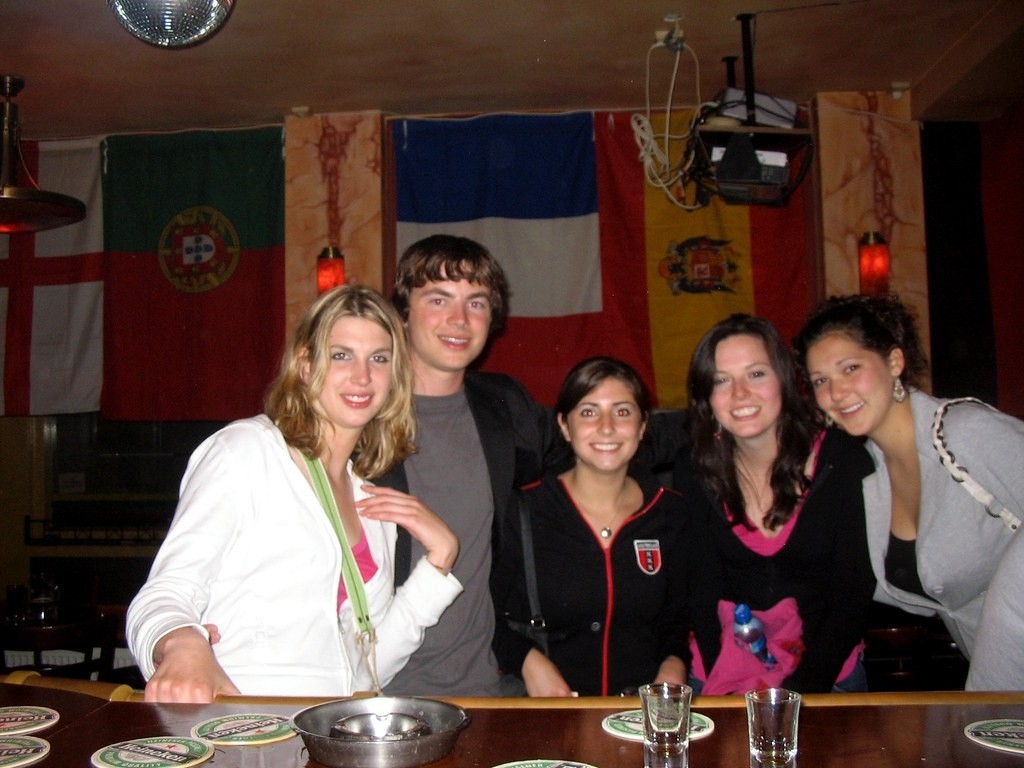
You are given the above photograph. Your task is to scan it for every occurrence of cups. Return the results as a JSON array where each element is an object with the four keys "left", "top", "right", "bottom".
[
  {"left": 745, "top": 687, "right": 801, "bottom": 768},
  {"left": 638, "top": 683, "right": 692, "bottom": 768}
]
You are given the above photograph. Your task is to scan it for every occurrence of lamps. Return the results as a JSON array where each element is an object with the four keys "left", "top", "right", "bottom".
[{"left": 0, "top": 75, "right": 86, "bottom": 235}]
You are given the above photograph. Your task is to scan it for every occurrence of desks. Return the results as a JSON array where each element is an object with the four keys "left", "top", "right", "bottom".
[{"left": 0, "top": 668, "right": 1024, "bottom": 768}]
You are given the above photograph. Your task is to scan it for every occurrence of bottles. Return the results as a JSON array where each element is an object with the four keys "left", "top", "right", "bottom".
[{"left": 732, "top": 604, "right": 769, "bottom": 662}]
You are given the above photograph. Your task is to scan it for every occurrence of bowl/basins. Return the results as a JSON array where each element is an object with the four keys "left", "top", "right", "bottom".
[{"left": 289, "top": 695, "right": 471, "bottom": 768}]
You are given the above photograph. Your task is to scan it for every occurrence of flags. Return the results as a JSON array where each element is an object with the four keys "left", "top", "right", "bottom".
[
  {"left": 0, "top": 120, "right": 286, "bottom": 422},
  {"left": 383, "top": 105, "right": 825, "bottom": 413}
]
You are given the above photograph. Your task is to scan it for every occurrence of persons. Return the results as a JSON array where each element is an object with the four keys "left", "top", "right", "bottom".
[
  {"left": 354, "top": 233, "right": 555, "bottom": 696},
  {"left": 487, "top": 356, "right": 711, "bottom": 698},
  {"left": 793, "top": 292, "right": 1024, "bottom": 694},
  {"left": 673, "top": 313, "right": 869, "bottom": 699},
  {"left": 123, "top": 283, "right": 467, "bottom": 705}
]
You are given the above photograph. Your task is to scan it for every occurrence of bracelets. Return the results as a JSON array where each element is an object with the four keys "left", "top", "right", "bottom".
[{"left": 429, "top": 561, "right": 455, "bottom": 573}]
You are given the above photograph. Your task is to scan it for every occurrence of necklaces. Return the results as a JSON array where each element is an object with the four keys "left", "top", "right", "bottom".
[
  {"left": 571, "top": 472, "right": 633, "bottom": 540},
  {"left": 732, "top": 443, "right": 775, "bottom": 516}
]
